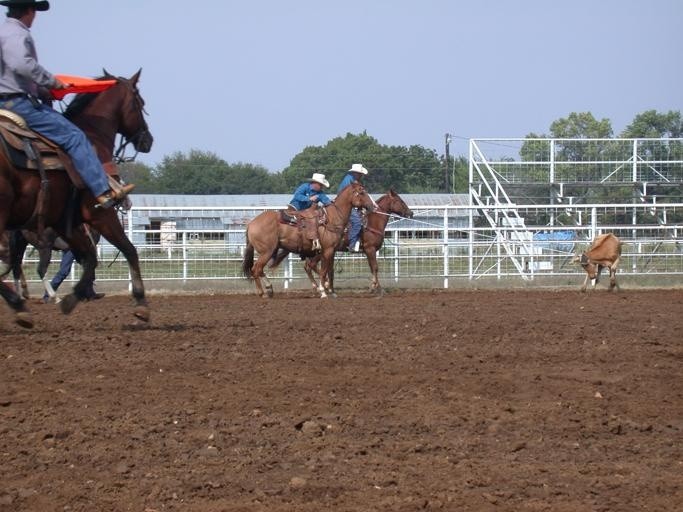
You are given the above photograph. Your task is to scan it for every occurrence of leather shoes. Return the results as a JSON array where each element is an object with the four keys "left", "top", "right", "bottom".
[
  {"left": 98, "top": 184, "right": 135, "bottom": 208},
  {"left": 90, "top": 293, "right": 104, "bottom": 300}
]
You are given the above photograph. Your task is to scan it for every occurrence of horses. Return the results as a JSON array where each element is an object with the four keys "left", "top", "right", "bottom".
[
  {"left": 299, "top": 187, "right": 416, "bottom": 297},
  {"left": 239, "top": 180, "right": 379, "bottom": 300},
  {"left": 0, "top": 67, "right": 154, "bottom": 326},
  {"left": 569, "top": 231, "right": 628, "bottom": 292}
]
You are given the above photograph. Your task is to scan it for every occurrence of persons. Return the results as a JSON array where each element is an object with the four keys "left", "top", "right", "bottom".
[
  {"left": 335, "top": 163, "right": 369, "bottom": 252},
  {"left": 287, "top": 173, "right": 333, "bottom": 210},
  {"left": 0, "top": 0, "right": 136, "bottom": 209},
  {"left": 39, "top": 239, "right": 108, "bottom": 304}
]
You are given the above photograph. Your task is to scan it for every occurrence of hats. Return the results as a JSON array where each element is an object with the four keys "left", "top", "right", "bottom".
[
  {"left": 1, "top": 0, "right": 50, "bottom": 12},
  {"left": 305, "top": 174, "right": 330, "bottom": 188},
  {"left": 348, "top": 164, "right": 368, "bottom": 174}
]
